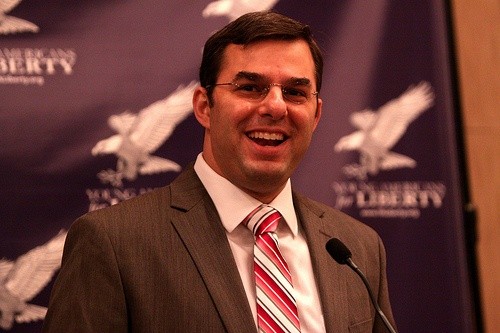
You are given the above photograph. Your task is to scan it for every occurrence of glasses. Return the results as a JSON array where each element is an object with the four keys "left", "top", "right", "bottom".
[{"left": 205, "top": 76, "right": 320, "bottom": 105}]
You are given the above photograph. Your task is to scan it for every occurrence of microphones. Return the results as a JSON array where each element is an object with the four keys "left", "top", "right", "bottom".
[{"left": 325, "top": 238, "right": 397, "bottom": 333}]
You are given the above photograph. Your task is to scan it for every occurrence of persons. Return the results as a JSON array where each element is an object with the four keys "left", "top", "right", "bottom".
[{"left": 43, "top": 11, "right": 397, "bottom": 333}]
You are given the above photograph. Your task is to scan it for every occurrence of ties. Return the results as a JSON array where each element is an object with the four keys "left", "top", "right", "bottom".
[{"left": 242, "top": 204, "right": 301, "bottom": 333}]
